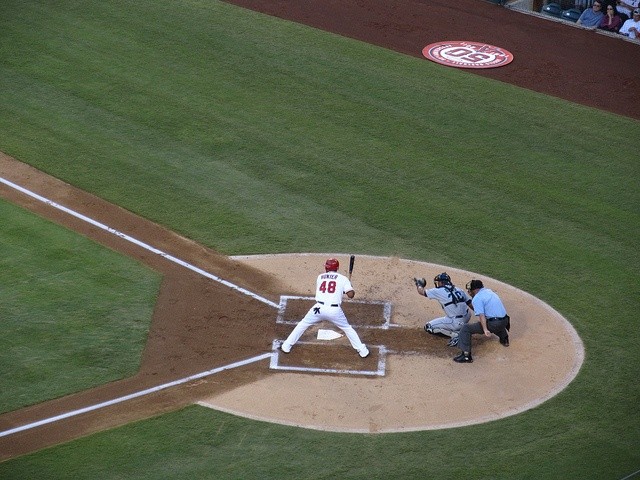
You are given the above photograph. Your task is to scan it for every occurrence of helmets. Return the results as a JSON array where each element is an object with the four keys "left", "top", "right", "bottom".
[
  {"left": 325, "top": 258, "right": 339, "bottom": 272},
  {"left": 434, "top": 272, "right": 451, "bottom": 288},
  {"left": 466, "top": 280, "right": 483, "bottom": 290}
]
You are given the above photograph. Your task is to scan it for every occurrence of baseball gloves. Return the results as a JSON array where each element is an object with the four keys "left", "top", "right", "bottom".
[{"left": 414, "top": 277, "right": 426, "bottom": 287}]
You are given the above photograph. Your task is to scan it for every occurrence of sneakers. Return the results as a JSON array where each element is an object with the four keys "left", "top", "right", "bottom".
[
  {"left": 447, "top": 337, "right": 459, "bottom": 347},
  {"left": 454, "top": 353, "right": 473, "bottom": 363},
  {"left": 275, "top": 340, "right": 290, "bottom": 353},
  {"left": 500, "top": 338, "right": 509, "bottom": 347},
  {"left": 360, "top": 344, "right": 369, "bottom": 356}
]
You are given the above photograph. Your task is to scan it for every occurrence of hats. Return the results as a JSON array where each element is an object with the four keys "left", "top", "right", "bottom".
[{"left": 633, "top": 8, "right": 640, "bottom": 15}]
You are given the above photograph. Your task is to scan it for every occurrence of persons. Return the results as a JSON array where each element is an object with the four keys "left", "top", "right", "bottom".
[
  {"left": 414, "top": 272, "right": 474, "bottom": 347},
  {"left": 618, "top": 8, "right": 640, "bottom": 40},
  {"left": 454, "top": 280, "right": 510, "bottom": 363},
  {"left": 576, "top": 0, "right": 604, "bottom": 29},
  {"left": 281, "top": 258, "right": 370, "bottom": 358},
  {"left": 600, "top": 2, "right": 622, "bottom": 33},
  {"left": 614, "top": 0, "right": 640, "bottom": 22}
]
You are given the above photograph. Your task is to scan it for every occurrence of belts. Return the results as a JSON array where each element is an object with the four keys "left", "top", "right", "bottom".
[
  {"left": 456, "top": 309, "right": 468, "bottom": 318},
  {"left": 486, "top": 314, "right": 508, "bottom": 321},
  {"left": 318, "top": 301, "right": 338, "bottom": 306}
]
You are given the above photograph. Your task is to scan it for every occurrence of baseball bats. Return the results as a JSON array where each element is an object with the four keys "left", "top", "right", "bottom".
[{"left": 348, "top": 255, "right": 355, "bottom": 282}]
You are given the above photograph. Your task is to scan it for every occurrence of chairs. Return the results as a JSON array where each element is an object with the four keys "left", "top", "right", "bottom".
[
  {"left": 541, "top": 3, "right": 562, "bottom": 18},
  {"left": 562, "top": 8, "right": 581, "bottom": 22}
]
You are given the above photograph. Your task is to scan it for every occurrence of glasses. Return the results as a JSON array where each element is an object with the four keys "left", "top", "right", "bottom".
[
  {"left": 593, "top": 5, "right": 601, "bottom": 7},
  {"left": 607, "top": 8, "right": 613, "bottom": 10}
]
